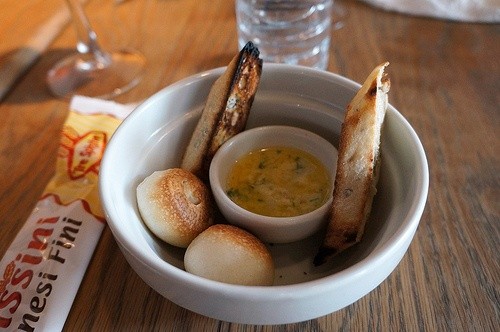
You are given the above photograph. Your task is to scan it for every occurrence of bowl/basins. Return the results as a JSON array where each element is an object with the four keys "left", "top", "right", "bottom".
[
  {"left": 210, "top": 124, "right": 341, "bottom": 246},
  {"left": 96, "top": 62, "right": 430, "bottom": 325}
]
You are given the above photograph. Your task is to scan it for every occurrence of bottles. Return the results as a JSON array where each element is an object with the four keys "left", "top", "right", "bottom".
[{"left": 236, "top": 0, "right": 333, "bottom": 71}]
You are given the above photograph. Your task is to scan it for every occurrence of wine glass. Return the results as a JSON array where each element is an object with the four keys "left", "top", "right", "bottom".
[{"left": 45, "top": 0, "right": 150, "bottom": 102}]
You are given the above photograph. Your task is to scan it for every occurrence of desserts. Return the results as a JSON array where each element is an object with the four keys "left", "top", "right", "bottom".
[
  {"left": 136, "top": 168, "right": 217, "bottom": 247},
  {"left": 184, "top": 224, "right": 274, "bottom": 287}
]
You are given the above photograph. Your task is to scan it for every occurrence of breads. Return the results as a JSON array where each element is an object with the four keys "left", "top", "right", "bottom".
[
  {"left": 313, "top": 61, "right": 390, "bottom": 266},
  {"left": 180, "top": 41, "right": 263, "bottom": 182}
]
